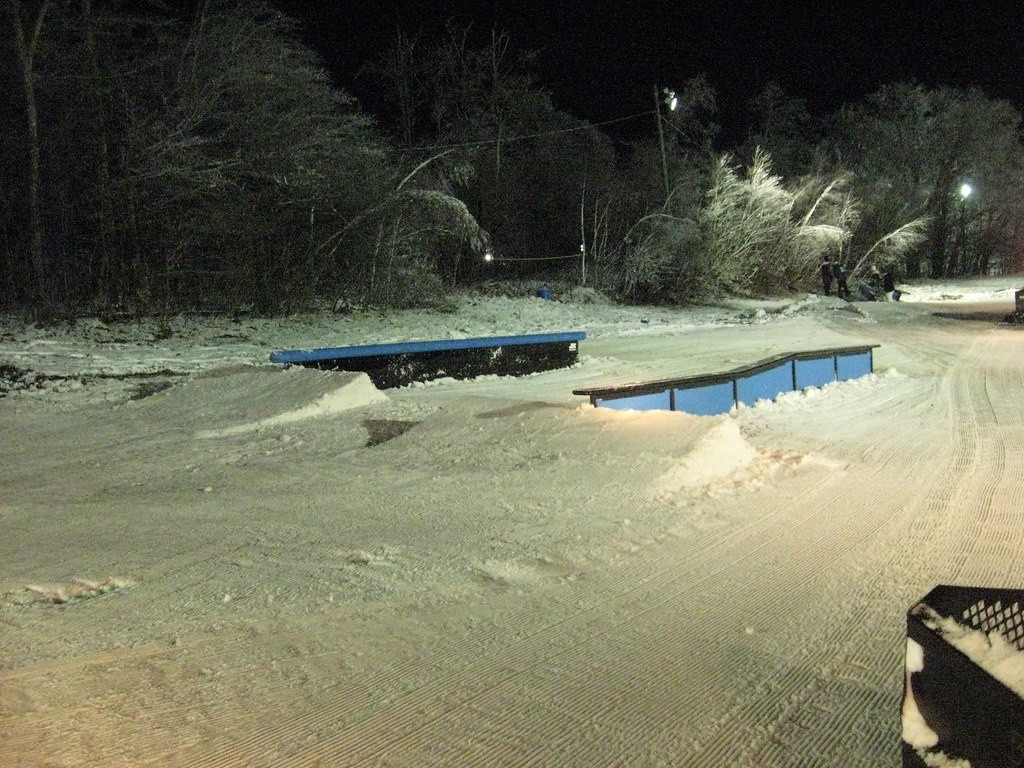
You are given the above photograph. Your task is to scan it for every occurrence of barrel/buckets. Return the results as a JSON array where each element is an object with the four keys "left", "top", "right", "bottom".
[
  {"left": 892, "top": 290, "right": 902, "bottom": 301},
  {"left": 538, "top": 288, "right": 553, "bottom": 300}
]
